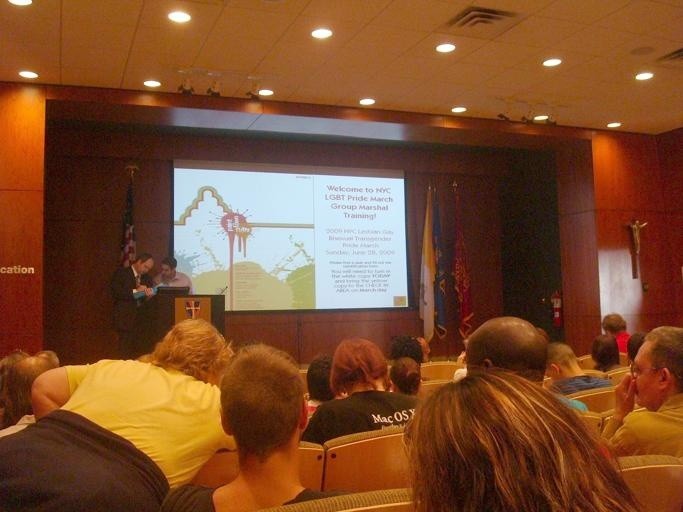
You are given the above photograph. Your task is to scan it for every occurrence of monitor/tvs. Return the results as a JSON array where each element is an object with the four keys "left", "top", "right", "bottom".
[{"left": 157, "top": 286, "right": 190, "bottom": 294}]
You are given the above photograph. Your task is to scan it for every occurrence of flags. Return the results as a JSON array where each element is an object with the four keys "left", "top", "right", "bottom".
[
  {"left": 121, "top": 168, "right": 140, "bottom": 272},
  {"left": 454, "top": 182, "right": 474, "bottom": 342},
  {"left": 420, "top": 186, "right": 436, "bottom": 350},
  {"left": 436, "top": 184, "right": 447, "bottom": 344}
]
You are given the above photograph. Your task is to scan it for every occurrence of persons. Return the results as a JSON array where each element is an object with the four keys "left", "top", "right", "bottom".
[
  {"left": 399, "top": 368, "right": 654, "bottom": 512},
  {"left": 601, "top": 327, "right": 683, "bottom": 457},
  {"left": 1, "top": 316, "right": 237, "bottom": 510},
  {"left": 113, "top": 253, "right": 190, "bottom": 360},
  {"left": 1, "top": 315, "right": 646, "bottom": 448},
  {"left": 160, "top": 341, "right": 351, "bottom": 512}
]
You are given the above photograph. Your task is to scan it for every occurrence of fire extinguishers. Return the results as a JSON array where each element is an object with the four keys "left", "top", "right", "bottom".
[{"left": 552, "top": 288, "right": 562, "bottom": 328}]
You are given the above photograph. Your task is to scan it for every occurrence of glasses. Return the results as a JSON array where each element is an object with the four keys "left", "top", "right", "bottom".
[{"left": 630, "top": 361, "right": 657, "bottom": 379}]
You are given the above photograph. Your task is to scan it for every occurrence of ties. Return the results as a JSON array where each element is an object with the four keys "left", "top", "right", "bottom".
[{"left": 136, "top": 275, "right": 139, "bottom": 287}]
